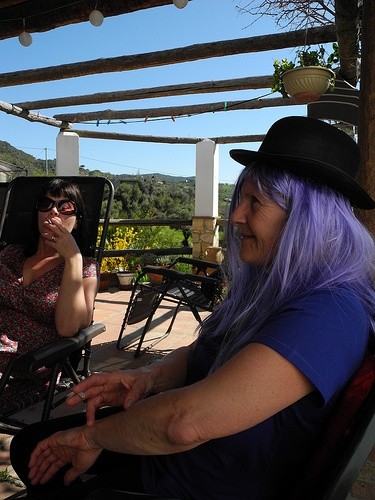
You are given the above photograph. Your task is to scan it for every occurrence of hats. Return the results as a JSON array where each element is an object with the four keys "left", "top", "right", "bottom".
[{"left": 229, "top": 116, "right": 375, "bottom": 210}]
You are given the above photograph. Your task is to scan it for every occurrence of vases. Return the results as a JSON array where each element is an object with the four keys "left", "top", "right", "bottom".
[
  {"left": 100, "top": 277, "right": 110, "bottom": 290},
  {"left": 116, "top": 273, "right": 134, "bottom": 285}
]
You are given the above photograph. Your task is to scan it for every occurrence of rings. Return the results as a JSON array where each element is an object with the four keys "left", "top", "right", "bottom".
[{"left": 51, "top": 235, "right": 55, "bottom": 241}]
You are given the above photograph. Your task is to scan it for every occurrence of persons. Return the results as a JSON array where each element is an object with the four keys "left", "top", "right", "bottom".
[
  {"left": 0, "top": 178, "right": 101, "bottom": 420},
  {"left": 65, "top": 116, "right": 375, "bottom": 500}
]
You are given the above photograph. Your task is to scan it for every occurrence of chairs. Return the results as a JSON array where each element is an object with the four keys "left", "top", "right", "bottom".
[{"left": 0, "top": 174, "right": 375, "bottom": 500}]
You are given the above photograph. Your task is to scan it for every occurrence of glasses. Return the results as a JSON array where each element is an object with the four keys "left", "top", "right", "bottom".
[{"left": 36, "top": 195, "right": 78, "bottom": 216}]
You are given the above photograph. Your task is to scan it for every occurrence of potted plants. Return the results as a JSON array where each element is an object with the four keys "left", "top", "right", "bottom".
[
  {"left": 139, "top": 250, "right": 172, "bottom": 284},
  {"left": 271, "top": 45, "right": 337, "bottom": 104}
]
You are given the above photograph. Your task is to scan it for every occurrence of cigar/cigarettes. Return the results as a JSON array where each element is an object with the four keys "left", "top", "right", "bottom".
[
  {"left": 44, "top": 217, "right": 53, "bottom": 224},
  {"left": 69, "top": 383, "right": 85, "bottom": 399}
]
